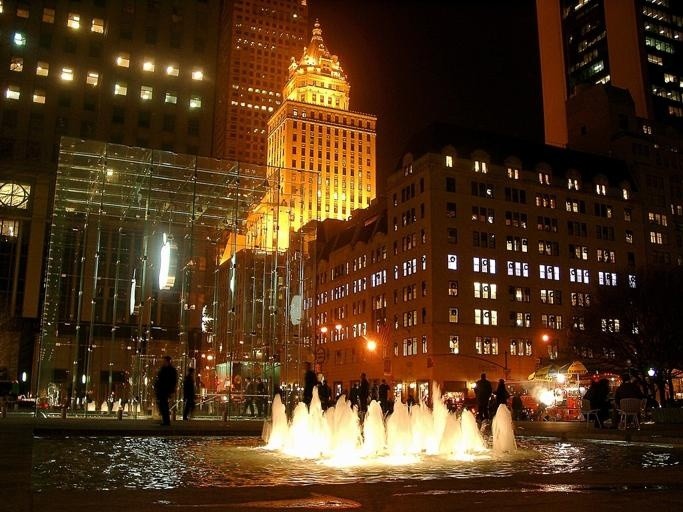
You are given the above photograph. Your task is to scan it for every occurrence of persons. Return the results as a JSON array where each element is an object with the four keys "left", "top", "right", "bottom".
[
  {"left": 614, "top": 372, "right": 641, "bottom": 400},
  {"left": 151, "top": 355, "right": 178, "bottom": 424},
  {"left": 473, "top": 372, "right": 489, "bottom": 419},
  {"left": 181, "top": 366, "right": 197, "bottom": 421},
  {"left": 583, "top": 377, "right": 615, "bottom": 431},
  {"left": 491, "top": 376, "right": 509, "bottom": 405},
  {"left": 241, "top": 361, "right": 416, "bottom": 421},
  {"left": 511, "top": 393, "right": 524, "bottom": 420}
]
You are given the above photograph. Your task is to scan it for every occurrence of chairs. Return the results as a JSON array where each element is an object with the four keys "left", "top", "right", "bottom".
[{"left": 577, "top": 397, "right": 646, "bottom": 433}]
